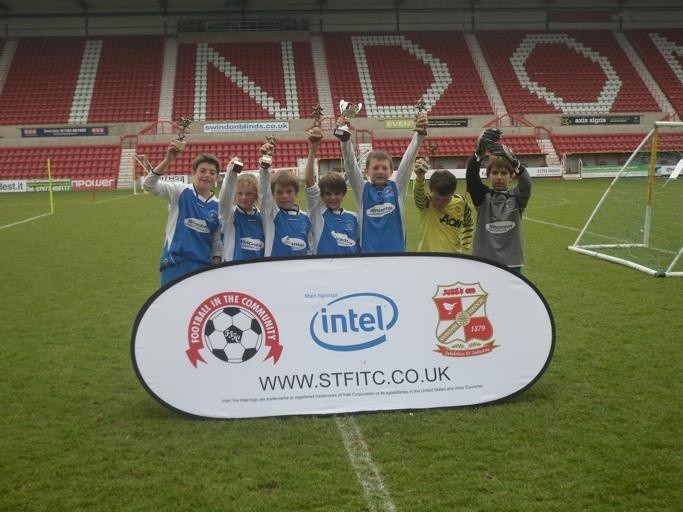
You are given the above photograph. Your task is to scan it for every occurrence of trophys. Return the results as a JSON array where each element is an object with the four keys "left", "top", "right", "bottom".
[
  {"left": 230, "top": 156, "right": 243, "bottom": 174},
  {"left": 310, "top": 104, "right": 324, "bottom": 141},
  {"left": 177, "top": 116, "right": 193, "bottom": 151},
  {"left": 333, "top": 99, "right": 362, "bottom": 142},
  {"left": 414, "top": 144, "right": 436, "bottom": 173},
  {"left": 414, "top": 97, "right": 427, "bottom": 136},
  {"left": 259, "top": 134, "right": 278, "bottom": 169},
  {"left": 489, "top": 154, "right": 502, "bottom": 162}
]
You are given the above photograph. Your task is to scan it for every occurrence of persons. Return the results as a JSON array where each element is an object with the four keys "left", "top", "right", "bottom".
[
  {"left": 305, "top": 128, "right": 359, "bottom": 255},
  {"left": 413, "top": 159, "right": 474, "bottom": 254},
  {"left": 258, "top": 143, "right": 312, "bottom": 257},
  {"left": 466, "top": 128, "right": 532, "bottom": 274},
  {"left": 336, "top": 113, "right": 429, "bottom": 255},
  {"left": 142, "top": 141, "right": 223, "bottom": 291},
  {"left": 218, "top": 156, "right": 265, "bottom": 262}
]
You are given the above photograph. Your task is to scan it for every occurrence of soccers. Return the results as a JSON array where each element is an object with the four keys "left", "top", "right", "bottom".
[{"left": 205, "top": 306, "right": 264, "bottom": 363}]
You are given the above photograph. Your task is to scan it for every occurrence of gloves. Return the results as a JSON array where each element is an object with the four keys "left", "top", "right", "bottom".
[
  {"left": 475, "top": 128, "right": 503, "bottom": 158},
  {"left": 488, "top": 143, "right": 520, "bottom": 170}
]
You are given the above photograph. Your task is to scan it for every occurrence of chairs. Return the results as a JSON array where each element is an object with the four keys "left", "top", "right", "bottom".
[
  {"left": 171, "top": 40, "right": 321, "bottom": 122},
  {"left": 0, "top": 144, "right": 121, "bottom": 179},
  {"left": 548, "top": 131, "right": 683, "bottom": 155},
  {"left": 321, "top": 29, "right": 495, "bottom": 119},
  {"left": 133, "top": 136, "right": 344, "bottom": 177},
  {"left": 0, "top": 33, "right": 166, "bottom": 124},
  {"left": 475, "top": 28, "right": 661, "bottom": 116},
  {"left": 626, "top": 27, "right": 683, "bottom": 121},
  {"left": 372, "top": 135, "right": 542, "bottom": 157}
]
